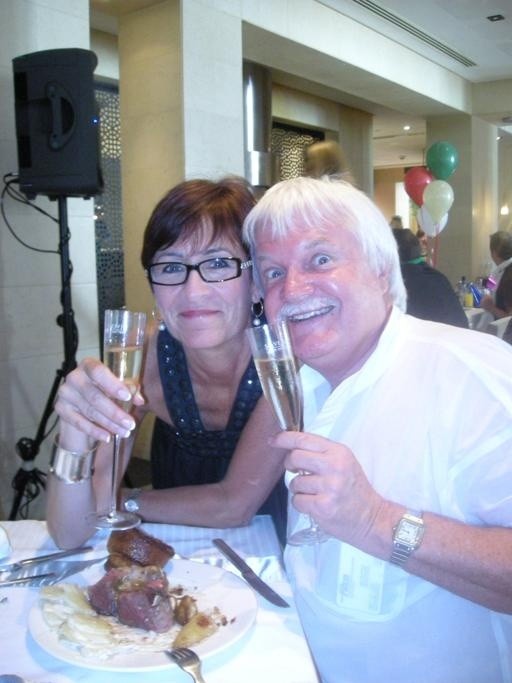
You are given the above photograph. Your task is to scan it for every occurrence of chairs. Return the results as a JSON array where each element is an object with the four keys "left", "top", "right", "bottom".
[{"left": 487, "top": 315, "right": 512, "bottom": 345}]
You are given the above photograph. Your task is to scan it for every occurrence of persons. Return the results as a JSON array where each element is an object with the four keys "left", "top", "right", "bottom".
[
  {"left": 43, "top": 174, "right": 296, "bottom": 552},
  {"left": 394, "top": 226, "right": 470, "bottom": 332},
  {"left": 476, "top": 231, "right": 511, "bottom": 346},
  {"left": 239, "top": 174, "right": 511, "bottom": 683}
]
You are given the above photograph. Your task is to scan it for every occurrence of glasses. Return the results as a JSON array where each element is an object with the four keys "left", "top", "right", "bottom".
[{"left": 145, "top": 256, "right": 256, "bottom": 286}]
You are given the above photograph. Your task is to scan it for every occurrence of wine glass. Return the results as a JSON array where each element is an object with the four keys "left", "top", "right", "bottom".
[
  {"left": 83, "top": 308, "right": 147, "bottom": 530},
  {"left": 245, "top": 318, "right": 331, "bottom": 547}
]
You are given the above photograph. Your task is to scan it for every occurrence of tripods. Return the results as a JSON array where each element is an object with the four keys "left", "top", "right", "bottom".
[{"left": 9, "top": 202, "right": 80, "bottom": 519}]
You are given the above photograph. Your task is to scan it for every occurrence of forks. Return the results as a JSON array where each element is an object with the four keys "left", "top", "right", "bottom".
[{"left": 164, "top": 646, "right": 206, "bottom": 683}]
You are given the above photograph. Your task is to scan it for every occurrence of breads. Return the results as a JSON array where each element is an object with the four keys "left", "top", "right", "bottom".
[{"left": 39, "top": 581, "right": 120, "bottom": 660}]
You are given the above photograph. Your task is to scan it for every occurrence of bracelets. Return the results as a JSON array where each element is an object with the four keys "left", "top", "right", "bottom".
[{"left": 47, "top": 431, "right": 98, "bottom": 482}]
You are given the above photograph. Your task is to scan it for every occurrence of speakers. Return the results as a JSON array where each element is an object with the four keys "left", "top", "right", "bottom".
[{"left": 12, "top": 48, "right": 107, "bottom": 200}]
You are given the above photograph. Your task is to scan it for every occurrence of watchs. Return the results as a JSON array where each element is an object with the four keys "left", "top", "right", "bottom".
[
  {"left": 391, "top": 508, "right": 425, "bottom": 572},
  {"left": 124, "top": 485, "right": 142, "bottom": 513}
]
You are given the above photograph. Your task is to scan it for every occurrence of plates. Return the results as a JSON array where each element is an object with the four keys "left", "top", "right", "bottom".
[{"left": 27, "top": 555, "right": 258, "bottom": 672}]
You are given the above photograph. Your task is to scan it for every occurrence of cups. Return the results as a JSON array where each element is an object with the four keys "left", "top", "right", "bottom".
[{"left": 464, "top": 287, "right": 473, "bottom": 308}]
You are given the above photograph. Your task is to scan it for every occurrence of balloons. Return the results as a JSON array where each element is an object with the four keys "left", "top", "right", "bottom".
[
  {"left": 421, "top": 178, "right": 455, "bottom": 225},
  {"left": 414, "top": 205, "right": 449, "bottom": 236},
  {"left": 405, "top": 166, "right": 434, "bottom": 208},
  {"left": 425, "top": 139, "right": 459, "bottom": 181}
]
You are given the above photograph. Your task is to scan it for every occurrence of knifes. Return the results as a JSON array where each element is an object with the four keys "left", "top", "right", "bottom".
[
  {"left": 0, "top": 546, "right": 93, "bottom": 575},
  {"left": 212, "top": 538, "right": 290, "bottom": 608}
]
